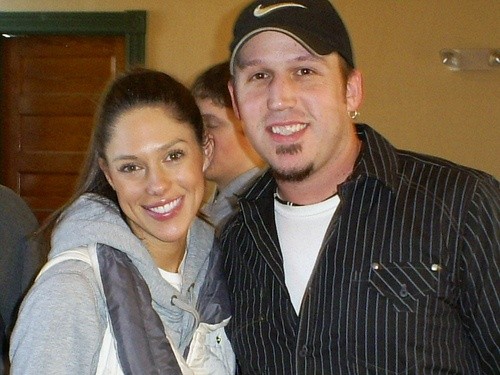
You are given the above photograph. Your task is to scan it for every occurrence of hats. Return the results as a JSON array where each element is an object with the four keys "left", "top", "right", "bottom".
[{"left": 229, "top": 0, "right": 354, "bottom": 76}]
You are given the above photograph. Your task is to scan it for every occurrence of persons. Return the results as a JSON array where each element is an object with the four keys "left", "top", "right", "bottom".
[
  {"left": 190, "top": 62, "right": 269, "bottom": 226},
  {"left": 217, "top": 0, "right": 500, "bottom": 375},
  {"left": 0, "top": 184, "right": 47, "bottom": 375},
  {"left": 9, "top": 70, "right": 216, "bottom": 374}
]
